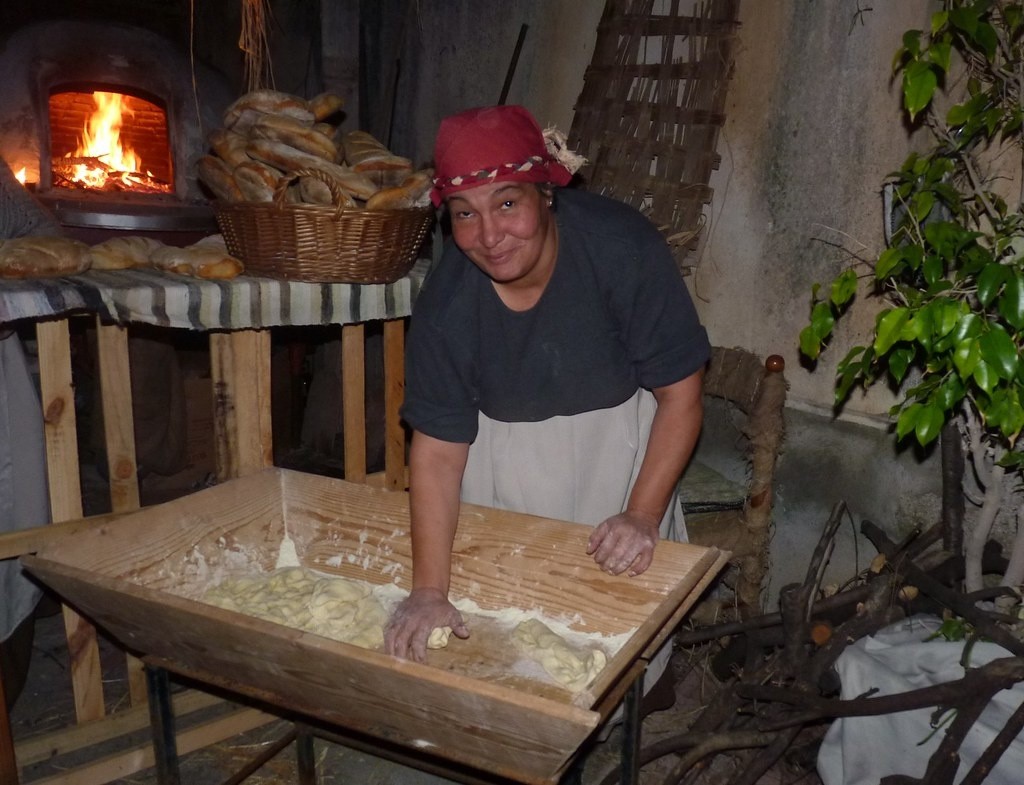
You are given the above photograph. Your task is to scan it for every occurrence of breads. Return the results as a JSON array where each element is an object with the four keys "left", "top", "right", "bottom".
[{"left": 196, "top": 90, "right": 432, "bottom": 213}]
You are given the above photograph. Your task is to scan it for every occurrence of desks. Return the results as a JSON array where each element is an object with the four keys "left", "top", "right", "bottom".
[{"left": 141, "top": 660, "right": 644, "bottom": 785}]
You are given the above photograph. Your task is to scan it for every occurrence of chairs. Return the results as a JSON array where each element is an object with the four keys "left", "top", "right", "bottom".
[{"left": 676, "top": 348, "right": 782, "bottom": 612}]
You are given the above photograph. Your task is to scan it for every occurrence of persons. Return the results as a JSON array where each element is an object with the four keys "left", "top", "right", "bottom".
[
  {"left": 382, "top": 106, "right": 711, "bottom": 724},
  {"left": 1, "top": 151, "right": 72, "bottom": 714}
]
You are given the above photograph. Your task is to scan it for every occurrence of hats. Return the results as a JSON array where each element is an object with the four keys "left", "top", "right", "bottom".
[{"left": 424, "top": 106, "right": 573, "bottom": 206}]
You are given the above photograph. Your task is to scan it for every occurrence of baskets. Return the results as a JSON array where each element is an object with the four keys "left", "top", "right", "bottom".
[{"left": 208, "top": 168, "right": 438, "bottom": 284}]
